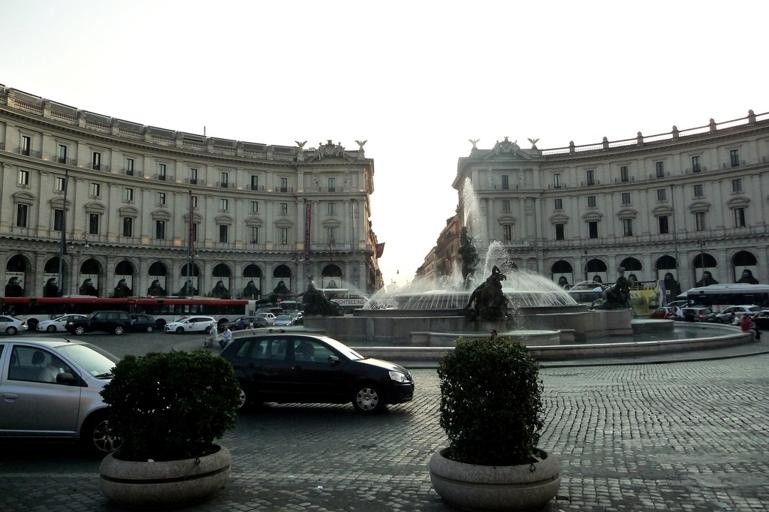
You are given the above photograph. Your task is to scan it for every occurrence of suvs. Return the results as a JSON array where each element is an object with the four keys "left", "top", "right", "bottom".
[{"left": 65, "top": 310, "right": 134, "bottom": 335}]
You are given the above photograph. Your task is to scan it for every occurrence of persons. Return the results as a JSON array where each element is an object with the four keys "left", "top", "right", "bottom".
[
  {"left": 730, "top": 312, "right": 763, "bottom": 342},
  {"left": 204, "top": 322, "right": 217, "bottom": 347},
  {"left": 218, "top": 326, "right": 233, "bottom": 348}
]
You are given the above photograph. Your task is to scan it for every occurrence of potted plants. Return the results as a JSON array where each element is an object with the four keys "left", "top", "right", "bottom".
[
  {"left": 96, "top": 347, "right": 245, "bottom": 508},
  {"left": 429, "top": 327, "right": 563, "bottom": 509}
]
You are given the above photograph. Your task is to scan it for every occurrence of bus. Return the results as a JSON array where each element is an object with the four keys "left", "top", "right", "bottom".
[
  {"left": 1, "top": 296, "right": 249, "bottom": 331},
  {"left": 687, "top": 284, "right": 769, "bottom": 308}
]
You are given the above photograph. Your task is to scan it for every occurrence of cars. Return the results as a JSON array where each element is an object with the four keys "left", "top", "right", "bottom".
[
  {"left": 0, "top": 338, "right": 124, "bottom": 455},
  {"left": 0, "top": 314, "right": 28, "bottom": 335},
  {"left": 655, "top": 300, "right": 769, "bottom": 328},
  {"left": 165, "top": 316, "right": 217, "bottom": 334},
  {"left": 37, "top": 314, "right": 86, "bottom": 333},
  {"left": 129, "top": 314, "right": 156, "bottom": 333},
  {"left": 221, "top": 335, "right": 414, "bottom": 414},
  {"left": 220, "top": 301, "right": 304, "bottom": 330}
]
